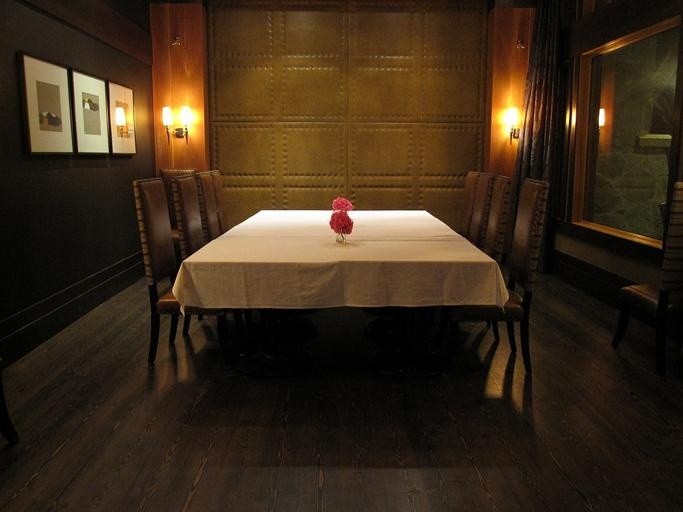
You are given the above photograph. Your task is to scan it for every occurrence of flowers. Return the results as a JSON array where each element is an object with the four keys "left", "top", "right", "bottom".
[{"left": 326, "top": 197, "right": 355, "bottom": 248}]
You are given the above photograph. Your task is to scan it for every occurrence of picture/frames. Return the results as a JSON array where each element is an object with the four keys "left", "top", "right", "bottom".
[
  {"left": 14, "top": 48, "right": 75, "bottom": 162},
  {"left": 106, "top": 78, "right": 136, "bottom": 161},
  {"left": 65, "top": 65, "right": 111, "bottom": 159}
]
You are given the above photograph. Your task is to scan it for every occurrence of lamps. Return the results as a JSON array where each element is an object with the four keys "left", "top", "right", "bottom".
[
  {"left": 499, "top": 104, "right": 523, "bottom": 146},
  {"left": 159, "top": 105, "right": 197, "bottom": 147}
]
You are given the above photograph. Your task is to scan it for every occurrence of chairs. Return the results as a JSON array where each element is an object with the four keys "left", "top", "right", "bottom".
[
  {"left": 610, "top": 180, "right": 683, "bottom": 376},
  {"left": 125, "top": 165, "right": 253, "bottom": 372},
  {"left": 413, "top": 168, "right": 550, "bottom": 377}
]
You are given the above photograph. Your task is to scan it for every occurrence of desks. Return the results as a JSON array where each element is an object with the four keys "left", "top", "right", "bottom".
[{"left": 160, "top": 207, "right": 510, "bottom": 389}]
